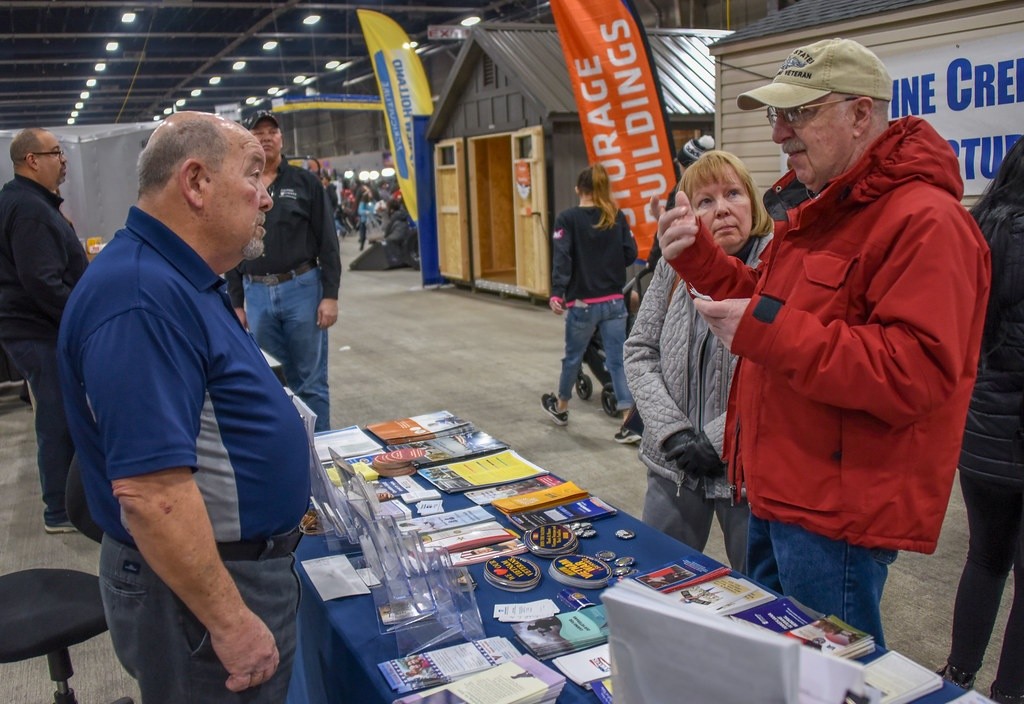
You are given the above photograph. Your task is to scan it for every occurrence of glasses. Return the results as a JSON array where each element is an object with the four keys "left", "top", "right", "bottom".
[
  {"left": 765, "top": 95, "right": 858, "bottom": 129},
  {"left": 24, "top": 150, "right": 63, "bottom": 161}
]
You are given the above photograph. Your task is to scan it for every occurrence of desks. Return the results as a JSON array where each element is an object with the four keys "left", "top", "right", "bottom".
[{"left": 286, "top": 410, "right": 1011, "bottom": 704}]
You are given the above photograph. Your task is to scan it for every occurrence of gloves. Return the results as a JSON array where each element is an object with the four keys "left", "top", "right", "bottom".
[{"left": 662, "top": 429, "right": 725, "bottom": 481}]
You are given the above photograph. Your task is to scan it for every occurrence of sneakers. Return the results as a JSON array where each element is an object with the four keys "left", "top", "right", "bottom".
[
  {"left": 541, "top": 393, "right": 568, "bottom": 426},
  {"left": 614, "top": 426, "right": 642, "bottom": 444},
  {"left": 44, "top": 521, "right": 78, "bottom": 533}
]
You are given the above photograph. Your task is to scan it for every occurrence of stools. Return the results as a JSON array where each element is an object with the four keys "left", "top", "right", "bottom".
[{"left": 0, "top": 567, "right": 133, "bottom": 704}]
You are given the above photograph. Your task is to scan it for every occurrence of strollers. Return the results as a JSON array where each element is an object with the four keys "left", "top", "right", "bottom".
[{"left": 561, "top": 268, "right": 657, "bottom": 416}]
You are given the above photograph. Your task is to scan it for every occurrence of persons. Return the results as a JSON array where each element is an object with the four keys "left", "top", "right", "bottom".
[
  {"left": 225, "top": 109, "right": 343, "bottom": 434},
  {"left": 623, "top": 148, "right": 775, "bottom": 576},
  {"left": 55, "top": 110, "right": 312, "bottom": 704},
  {"left": 647, "top": 135, "right": 715, "bottom": 271},
  {"left": 657, "top": 35, "right": 993, "bottom": 653},
  {"left": 324, "top": 176, "right": 346, "bottom": 239},
  {"left": 0, "top": 126, "right": 92, "bottom": 535},
  {"left": 339, "top": 181, "right": 402, "bottom": 231},
  {"left": 934, "top": 133, "right": 1024, "bottom": 704},
  {"left": 541, "top": 163, "right": 641, "bottom": 444},
  {"left": 629, "top": 266, "right": 655, "bottom": 316}
]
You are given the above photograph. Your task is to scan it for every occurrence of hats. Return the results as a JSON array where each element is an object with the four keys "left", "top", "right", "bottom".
[
  {"left": 678, "top": 134, "right": 714, "bottom": 168},
  {"left": 736, "top": 38, "right": 893, "bottom": 110},
  {"left": 241, "top": 103, "right": 279, "bottom": 131}
]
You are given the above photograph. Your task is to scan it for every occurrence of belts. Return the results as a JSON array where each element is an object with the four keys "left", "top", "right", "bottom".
[
  {"left": 214, "top": 530, "right": 304, "bottom": 561},
  {"left": 244, "top": 260, "right": 317, "bottom": 286}
]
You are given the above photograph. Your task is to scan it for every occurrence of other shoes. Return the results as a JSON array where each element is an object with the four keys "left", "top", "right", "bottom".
[
  {"left": 989, "top": 679, "right": 1024, "bottom": 704},
  {"left": 935, "top": 664, "right": 977, "bottom": 691}
]
